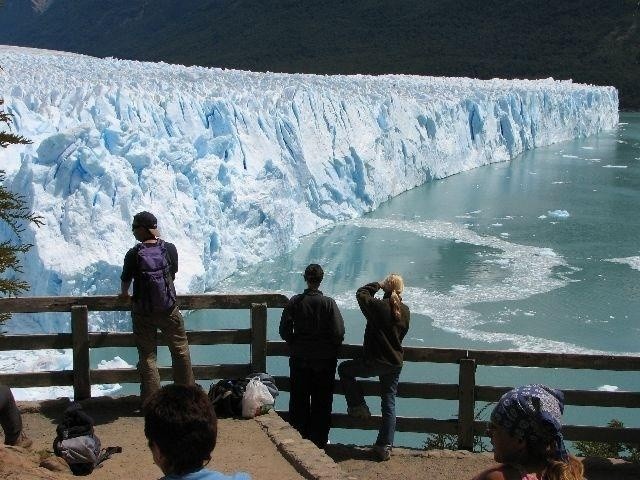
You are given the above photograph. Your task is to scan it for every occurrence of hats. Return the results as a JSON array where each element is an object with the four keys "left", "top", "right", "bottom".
[{"left": 134, "top": 212, "right": 162, "bottom": 238}]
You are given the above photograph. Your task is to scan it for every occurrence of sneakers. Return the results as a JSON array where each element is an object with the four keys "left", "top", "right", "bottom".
[
  {"left": 347, "top": 407, "right": 371, "bottom": 420},
  {"left": 371, "top": 444, "right": 393, "bottom": 461},
  {"left": 6, "top": 436, "right": 30, "bottom": 448}
]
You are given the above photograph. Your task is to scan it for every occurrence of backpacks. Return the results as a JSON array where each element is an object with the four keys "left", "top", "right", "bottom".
[{"left": 133, "top": 240, "right": 176, "bottom": 315}]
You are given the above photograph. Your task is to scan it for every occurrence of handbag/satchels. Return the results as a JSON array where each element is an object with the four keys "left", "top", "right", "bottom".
[
  {"left": 208, "top": 372, "right": 279, "bottom": 418},
  {"left": 54, "top": 398, "right": 100, "bottom": 476}
]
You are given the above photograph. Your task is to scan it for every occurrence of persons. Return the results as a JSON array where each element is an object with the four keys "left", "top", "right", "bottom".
[
  {"left": 119, "top": 211, "right": 202, "bottom": 401},
  {"left": 0, "top": 383, "right": 33, "bottom": 448},
  {"left": 472, "top": 384, "right": 587, "bottom": 480},
  {"left": 279, "top": 264, "right": 346, "bottom": 450},
  {"left": 338, "top": 274, "right": 411, "bottom": 459},
  {"left": 143, "top": 382, "right": 253, "bottom": 480}
]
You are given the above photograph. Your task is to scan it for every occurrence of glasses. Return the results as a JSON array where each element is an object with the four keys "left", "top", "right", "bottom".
[
  {"left": 132, "top": 224, "right": 139, "bottom": 228},
  {"left": 485, "top": 421, "right": 505, "bottom": 438}
]
